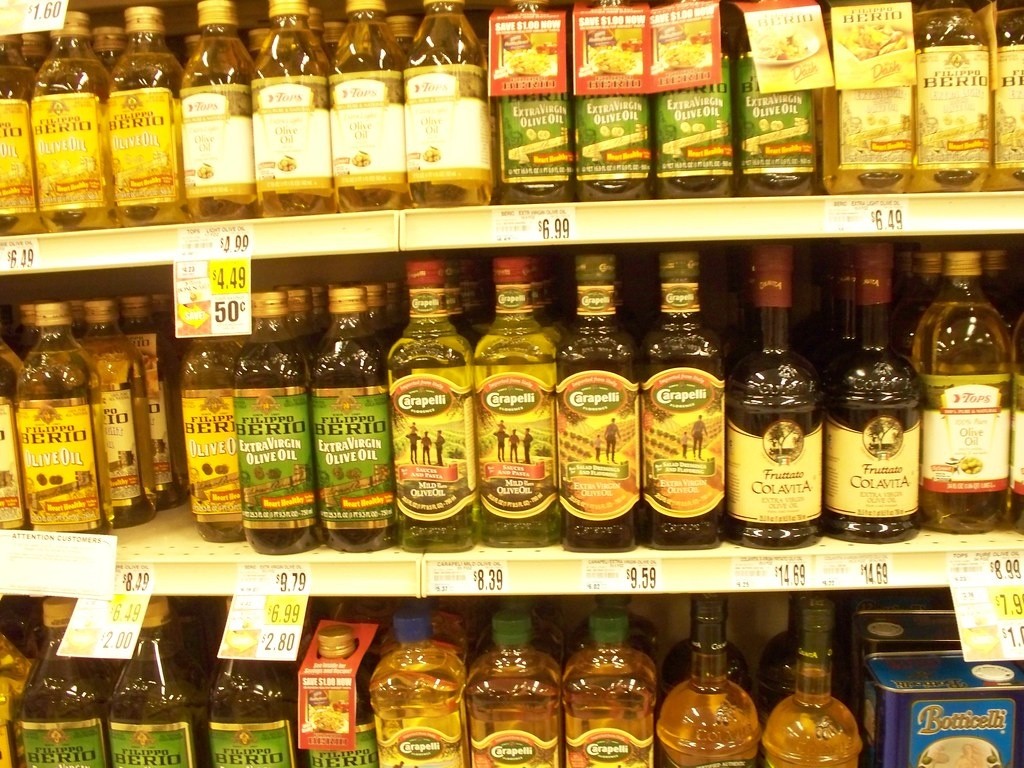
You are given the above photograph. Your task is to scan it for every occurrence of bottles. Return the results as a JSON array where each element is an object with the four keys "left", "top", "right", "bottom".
[{"left": 0, "top": 0, "right": 1024, "bottom": 768}]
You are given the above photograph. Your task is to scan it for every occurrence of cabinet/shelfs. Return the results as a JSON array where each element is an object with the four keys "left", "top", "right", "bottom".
[{"left": 0, "top": 0, "right": 1024, "bottom": 768}]
